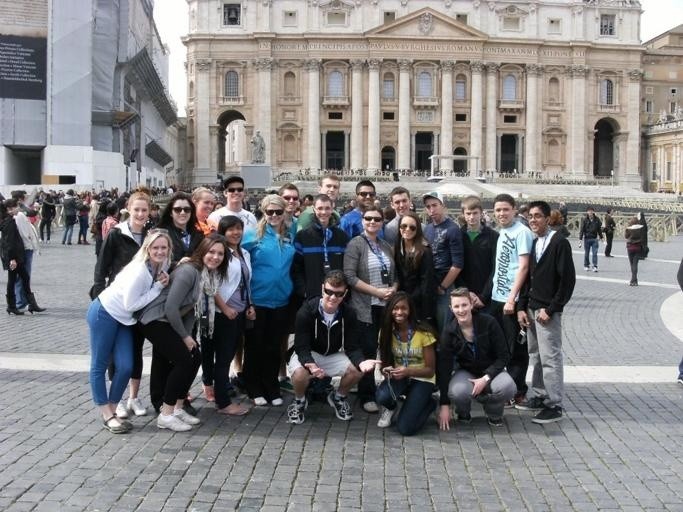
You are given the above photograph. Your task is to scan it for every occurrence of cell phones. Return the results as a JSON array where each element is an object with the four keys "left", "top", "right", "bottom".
[{"left": 385, "top": 369, "right": 393, "bottom": 373}]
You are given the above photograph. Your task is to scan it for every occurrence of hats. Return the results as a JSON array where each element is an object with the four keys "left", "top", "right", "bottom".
[
  {"left": 223, "top": 175, "right": 244, "bottom": 188},
  {"left": 421, "top": 191, "right": 443, "bottom": 203}
]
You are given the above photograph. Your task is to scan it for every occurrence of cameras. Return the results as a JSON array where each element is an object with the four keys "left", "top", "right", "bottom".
[{"left": 517, "top": 330, "right": 527, "bottom": 345}]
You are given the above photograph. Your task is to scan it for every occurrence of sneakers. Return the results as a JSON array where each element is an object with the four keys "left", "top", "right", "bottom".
[
  {"left": 101, "top": 373, "right": 354, "bottom": 435},
  {"left": 39, "top": 238, "right": 91, "bottom": 245},
  {"left": 349, "top": 382, "right": 562, "bottom": 427}
]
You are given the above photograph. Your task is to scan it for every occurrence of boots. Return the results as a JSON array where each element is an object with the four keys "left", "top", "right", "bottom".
[
  {"left": 25, "top": 292, "right": 47, "bottom": 314},
  {"left": 6, "top": 294, "right": 24, "bottom": 315}
]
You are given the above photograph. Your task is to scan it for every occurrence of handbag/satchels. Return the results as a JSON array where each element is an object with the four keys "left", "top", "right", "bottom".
[{"left": 642, "top": 245, "right": 649, "bottom": 258}]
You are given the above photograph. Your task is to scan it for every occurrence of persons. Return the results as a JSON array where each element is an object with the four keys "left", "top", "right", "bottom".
[
  {"left": 637, "top": 212, "right": 649, "bottom": 260},
  {"left": 1, "top": 172, "right": 575, "bottom": 437},
  {"left": 579, "top": 206, "right": 604, "bottom": 272},
  {"left": 676, "top": 259, "right": 683, "bottom": 386},
  {"left": 624, "top": 216, "right": 644, "bottom": 285},
  {"left": 604, "top": 208, "right": 616, "bottom": 257},
  {"left": 250, "top": 129, "right": 268, "bottom": 166}
]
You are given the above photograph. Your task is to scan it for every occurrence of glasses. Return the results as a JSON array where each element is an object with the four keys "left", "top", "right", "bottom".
[
  {"left": 527, "top": 213, "right": 546, "bottom": 220},
  {"left": 323, "top": 285, "right": 346, "bottom": 297},
  {"left": 148, "top": 188, "right": 417, "bottom": 233}
]
